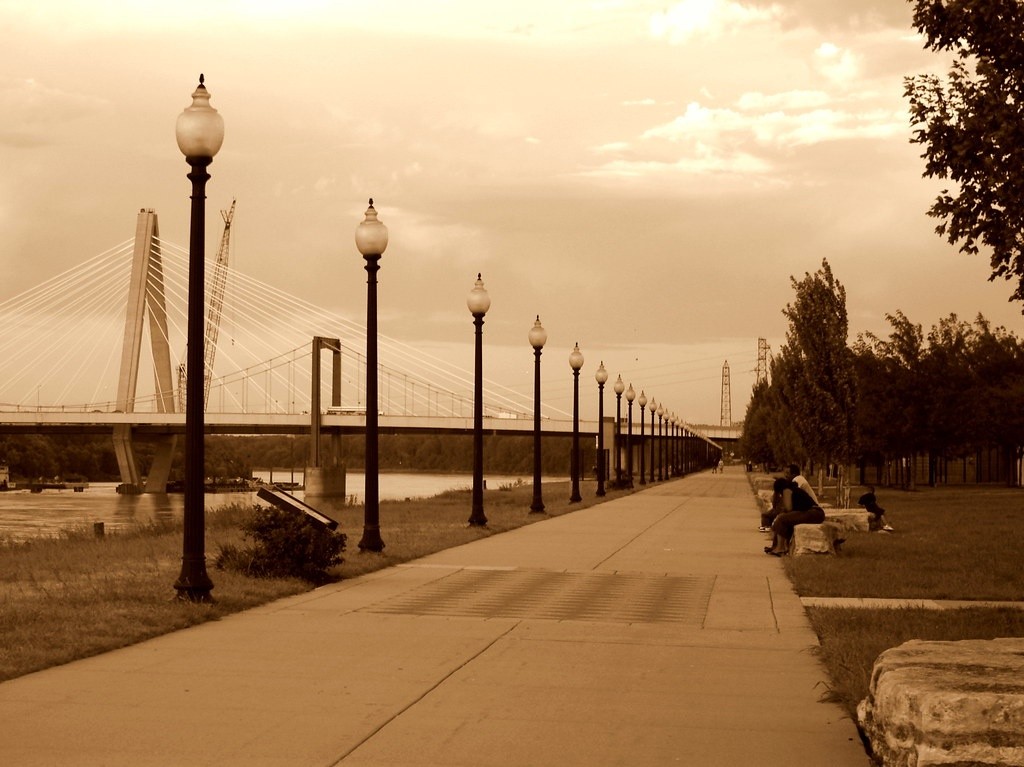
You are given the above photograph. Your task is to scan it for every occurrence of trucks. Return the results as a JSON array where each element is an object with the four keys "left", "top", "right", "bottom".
[
  {"left": 327, "top": 407, "right": 384, "bottom": 416},
  {"left": 492, "top": 412, "right": 518, "bottom": 419}
]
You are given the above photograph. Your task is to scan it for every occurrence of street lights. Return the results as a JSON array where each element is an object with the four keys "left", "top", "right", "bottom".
[
  {"left": 594, "top": 362, "right": 607, "bottom": 496},
  {"left": 467, "top": 272, "right": 493, "bottom": 530},
  {"left": 173, "top": 70, "right": 225, "bottom": 606},
  {"left": 649, "top": 395, "right": 655, "bottom": 483},
  {"left": 657, "top": 404, "right": 661, "bottom": 485},
  {"left": 638, "top": 391, "right": 647, "bottom": 485},
  {"left": 664, "top": 409, "right": 724, "bottom": 478},
  {"left": 528, "top": 315, "right": 549, "bottom": 515},
  {"left": 567, "top": 343, "right": 586, "bottom": 504},
  {"left": 355, "top": 195, "right": 386, "bottom": 552},
  {"left": 625, "top": 384, "right": 636, "bottom": 488},
  {"left": 613, "top": 374, "right": 624, "bottom": 488}
]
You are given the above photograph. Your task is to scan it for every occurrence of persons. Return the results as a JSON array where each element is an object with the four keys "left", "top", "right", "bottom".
[
  {"left": 858, "top": 485, "right": 893, "bottom": 530},
  {"left": 757, "top": 464, "right": 818, "bottom": 532},
  {"left": 746, "top": 460, "right": 753, "bottom": 472},
  {"left": 762, "top": 478, "right": 826, "bottom": 558},
  {"left": 711, "top": 458, "right": 723, "bottom": 474}
]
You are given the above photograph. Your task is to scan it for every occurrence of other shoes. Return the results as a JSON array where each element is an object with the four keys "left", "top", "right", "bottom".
[
  {"left": 771, "top": 549, "right": 786, "bottom": 557},
  {"left": 765, "top": 547, "right": 775, "bottom": 552},
  {"left": 883, "top": 525, "right": 894, "bottom": 530},
  {"left": 878, "top": 530, "right": 887, "bottom": 534},
  {"left": 758, "top": 526, "right": 772, "bottom": 532}
]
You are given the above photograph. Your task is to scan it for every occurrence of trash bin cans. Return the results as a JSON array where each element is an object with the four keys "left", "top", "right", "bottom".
[{"left": 747, "top": 464, "right": 752, "bottom": 472}]
